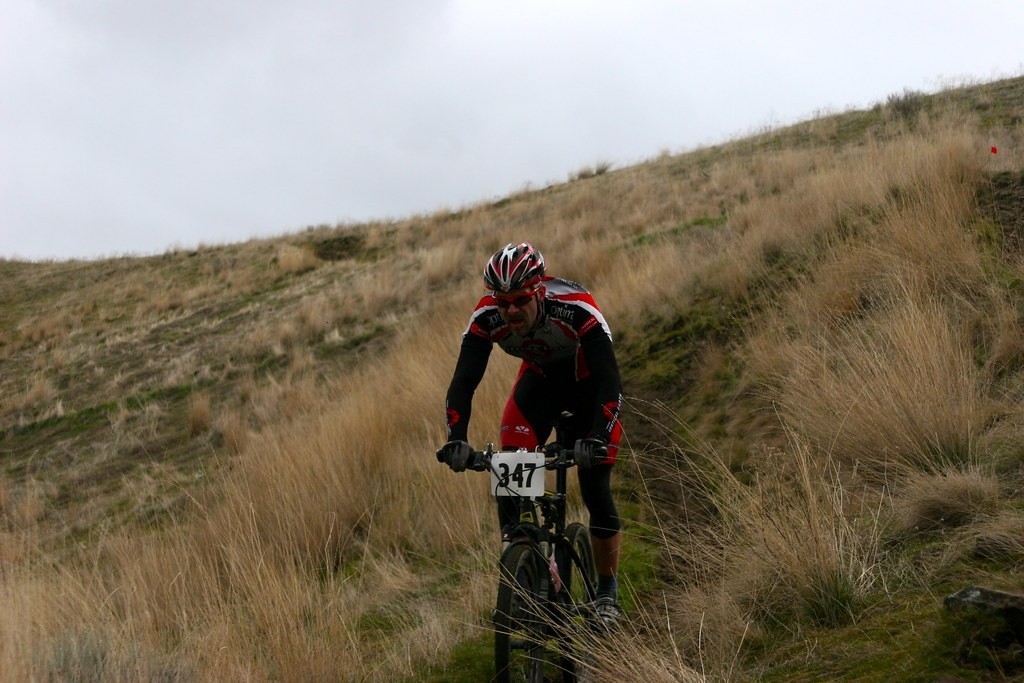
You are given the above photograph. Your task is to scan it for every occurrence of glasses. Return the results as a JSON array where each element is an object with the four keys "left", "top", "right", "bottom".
[{"left": 493, "top": 286, "right": 539, "bottom": 308}]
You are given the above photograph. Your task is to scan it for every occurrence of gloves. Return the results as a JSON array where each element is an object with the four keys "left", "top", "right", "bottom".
[
  {"left": 443, "top": 439, "right": 473, "bottom": 473},
  {"left": 574, "top": 436, "right": 607, "bottom": 467}
]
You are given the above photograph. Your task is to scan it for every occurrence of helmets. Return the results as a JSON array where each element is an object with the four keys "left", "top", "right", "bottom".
[{"left": 483, "top": 241, "right": 545, "bottom": 294}]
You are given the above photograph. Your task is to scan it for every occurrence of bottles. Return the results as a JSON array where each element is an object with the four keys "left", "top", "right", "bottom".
[{"left": 548, "top": 556, "right": 560, "bottom": 593}]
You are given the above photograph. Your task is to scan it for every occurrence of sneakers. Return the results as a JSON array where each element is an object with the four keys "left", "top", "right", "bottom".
[
  {"left": 590, "top": 594, "right": 622, "bottom": 632},
  {"left": 493, "top": 592, "right": 524, "bottom": 632}
]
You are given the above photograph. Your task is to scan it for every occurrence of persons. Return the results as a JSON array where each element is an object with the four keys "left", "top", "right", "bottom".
[{"left": 439, "top": 240, "right": 627, "bottom": 639}]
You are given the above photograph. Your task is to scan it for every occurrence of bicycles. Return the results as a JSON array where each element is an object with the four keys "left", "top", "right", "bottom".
[{"left": 435, "top": 442, "right": 610, "bottom": 683}]
